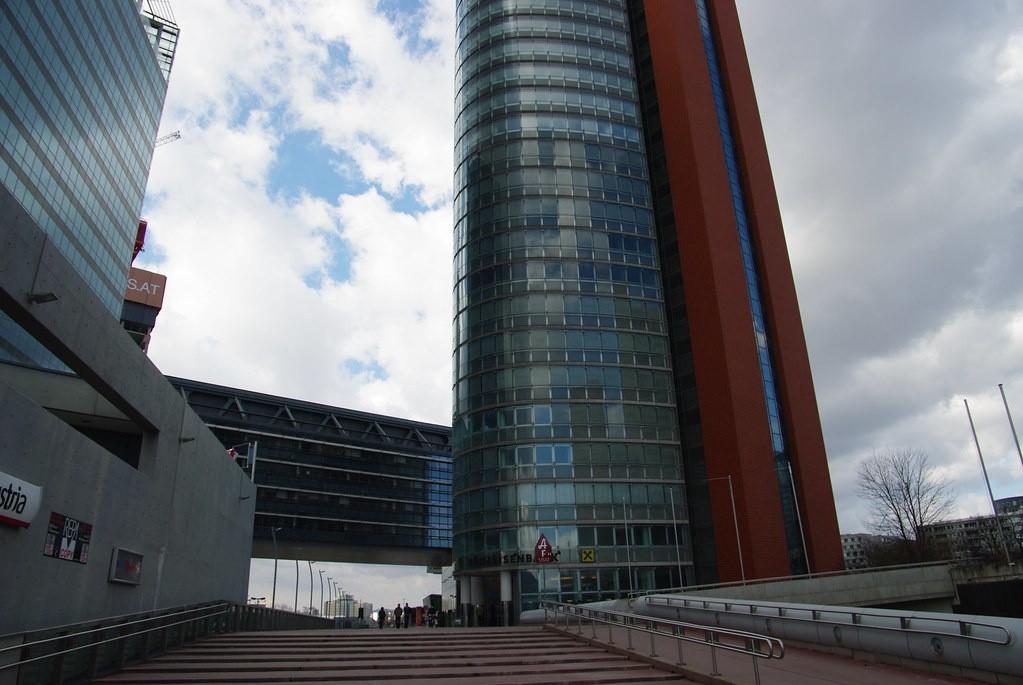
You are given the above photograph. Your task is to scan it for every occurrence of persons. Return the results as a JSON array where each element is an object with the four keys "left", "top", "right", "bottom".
[
  {"left": 404, "top": 603, "right": 410, "bottom": 628},
  {"left": 378, "top": 607, "right": 386, "bottom": 629},
  {"left": 428, "top": 605, "right": 437, "bottom": 627},
  {"left": 394, "top": 604, "right": 402, "bottom": 629}
]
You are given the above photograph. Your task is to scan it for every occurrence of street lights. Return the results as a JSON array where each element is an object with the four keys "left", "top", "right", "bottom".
[
  {"left": 338, "top": 587, "right": 357, "bottom": 618},
  {"left": 270, "top": 526, "right": 283, "bottom": 607},
  {"left": 319, "top": 569, "right": 325, "bottom": 617},
  {"left": 308, "top": 558, "right": 316, "bottom": 615},
  {"left": 293, "top": 546, "right": 304, "bottom": 614},
  {"left": 333, "top": 582, "right": 337, "bottom": 619},
  {"left": 328, "top": 578, "right": 333, "bottom": 617}
]
